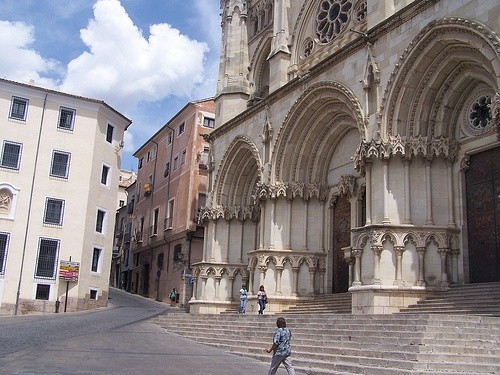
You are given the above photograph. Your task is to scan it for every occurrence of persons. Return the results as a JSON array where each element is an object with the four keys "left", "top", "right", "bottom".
[
  {"left": 257, "top": 285, "right": 269, "bottom": 314},
  {"left": 169, "top": 288, "right": 177, "bottom": 307},
  {"left": 238, "top": 284, "right": 248, "bottom": 314},
  {"left": 263, "top": 317, "right": 296, "bottom": 374}
]
